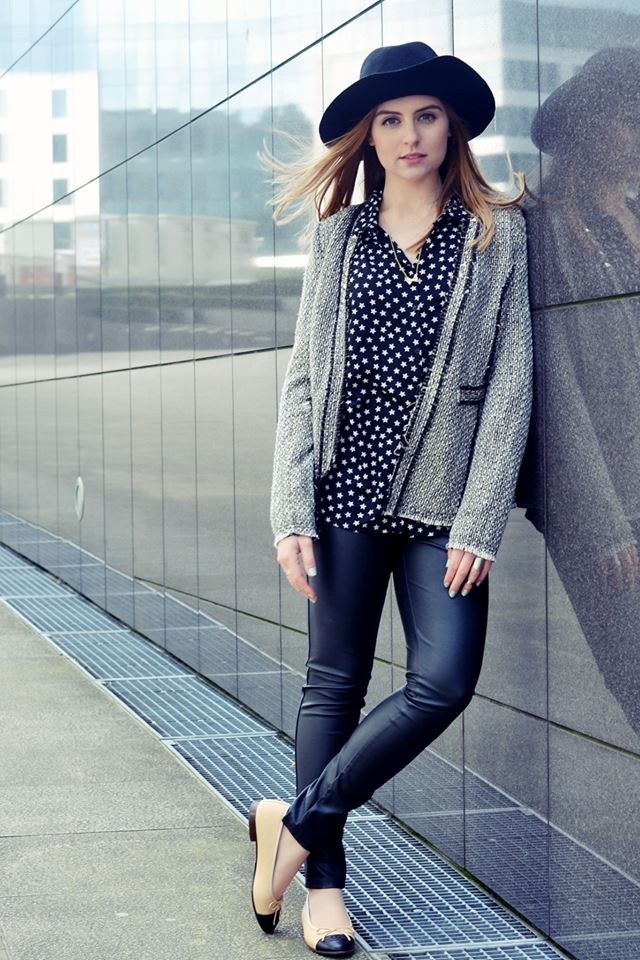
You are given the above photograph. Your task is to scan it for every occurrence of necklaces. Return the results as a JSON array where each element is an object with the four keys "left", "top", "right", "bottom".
[{"left": 381, "top": 224, "right": 423, "bottom": 282}]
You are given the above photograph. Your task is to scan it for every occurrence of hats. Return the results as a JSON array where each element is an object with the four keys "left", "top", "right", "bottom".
[
  {"left": 319, "top": 41, "right": 496, "bottom": 161},
  {"left": 530, "top": 48, "right": 640, "bottom": 159}
]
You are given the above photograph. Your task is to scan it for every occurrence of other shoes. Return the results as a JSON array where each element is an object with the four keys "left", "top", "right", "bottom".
[
  {"left": 301, "top": 895, "right": 356, "bottom": 956},
  {"left": 248, "top": 799, "right": 291, "bottom": 933}
]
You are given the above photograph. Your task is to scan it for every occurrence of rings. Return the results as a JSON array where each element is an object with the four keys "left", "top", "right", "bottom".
[
  {"left": 467, "top": 580, "right": 474, "bottom": 586},
  {"left": 285, "top": 571, "right": 291, "bottom": 576},
  {"left": 473, "top": 556, "right": 483, "bottom": 572}
]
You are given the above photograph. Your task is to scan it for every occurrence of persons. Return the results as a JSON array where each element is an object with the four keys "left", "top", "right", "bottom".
[
  {"left": 250, "top": 40, "right": 535, "bottom": 960},
  {"left": 518, "top": 48, "right": 640, "bottom": 739}
]
